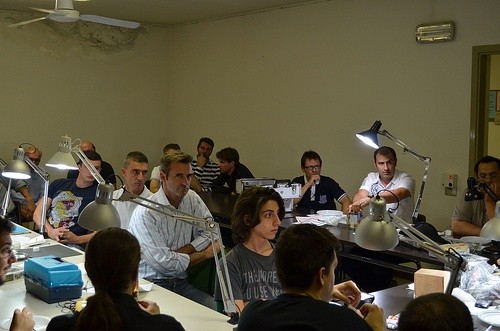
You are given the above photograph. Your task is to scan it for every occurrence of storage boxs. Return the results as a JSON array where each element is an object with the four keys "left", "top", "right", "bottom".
[
  {"left": 413, "top": 269, "right": 451, "bottom": 296},
  {"left": 24, "top": 254, "right": 83, "bottom": 304}
]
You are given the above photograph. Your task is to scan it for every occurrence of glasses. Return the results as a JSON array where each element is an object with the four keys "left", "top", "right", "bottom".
[
  {"left": 304, "top": 164, "right": 320, "bottom": 170},
  {"left": 0, "top": 244, "right": 15, "bottom": 259}
]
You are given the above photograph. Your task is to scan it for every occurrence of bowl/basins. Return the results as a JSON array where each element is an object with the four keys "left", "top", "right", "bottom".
[{"left": 317, "top": 210, "right": 344, "bottom": 221}]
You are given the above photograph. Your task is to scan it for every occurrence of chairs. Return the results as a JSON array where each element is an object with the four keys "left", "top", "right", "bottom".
[{"left": 187, "top": 247, "right": 226, "bottom": 296}]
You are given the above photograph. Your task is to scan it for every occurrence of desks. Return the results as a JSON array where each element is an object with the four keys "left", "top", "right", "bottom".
[
  {"left": 356, "top": 284, "right": 500, "bottom": 331},
  {"left": 276, "top": 210, "right": 448, "bottom": 281},
  {"left": 0, "top": 214, "right": 238, "bottom": 331}
]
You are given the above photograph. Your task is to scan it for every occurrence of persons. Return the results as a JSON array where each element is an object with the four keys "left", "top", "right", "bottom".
[
  {"left": 237, "top": 224, "right": 388, "bottom": 331},
  {"left": 10, "top": 135, "right": 255, "bottom": 312},
  {"left": 0, "top": 218, "right": 35, "bottom": 331},
  {"left": 46, "top": 227, "right": 185, "bottom": 331},
  {"left": 214, "top": 186, "right": 285, "bottom": 316},
  {"left": 343, "top": 146, "right": 416, "bottom": 292},
  {"left": 452, "top": 156, "right": 500, "bottom": 235},
  {"left": 398, "top": 293, "right": 474, "bottom": 331},
  {"left": 291, "top": 150, "right": 351, "bottom": 213}
]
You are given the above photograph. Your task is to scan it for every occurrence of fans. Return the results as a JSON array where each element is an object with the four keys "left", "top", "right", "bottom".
[{"left": 9, "top": 0, "right": 141, "bottom": 28}]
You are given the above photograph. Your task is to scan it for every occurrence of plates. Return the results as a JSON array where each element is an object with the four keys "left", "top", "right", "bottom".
[
  {"left": 0, "top": 315, "right": 53, "bottom": 331},
  {"left": 460, "top": 236, "right": 491, "bottom": 244}
]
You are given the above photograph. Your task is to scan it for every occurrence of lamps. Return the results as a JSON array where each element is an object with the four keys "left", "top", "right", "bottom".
[
  {"left": 356, "top": 121, "right": 431, "bottom": 221},
  {"left": 415, "top": 22, "right": 455, "bottom": 44},
  {"left": 77, "top": 182, "right": 238, "bottom": 325},
  {"left": 353, "top": 194, "right": 467, "bottom": 296},
  {"left": 46, "top": 135, "right": 105, "bottom": 234},
  {"left": 1, "top": 145, "right": 50, "bottom": 239}
]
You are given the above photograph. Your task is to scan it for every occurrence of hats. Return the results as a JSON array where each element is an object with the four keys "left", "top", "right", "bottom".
[{"left": 412, "top": 221, "right": 451, "bottom": 245}]
